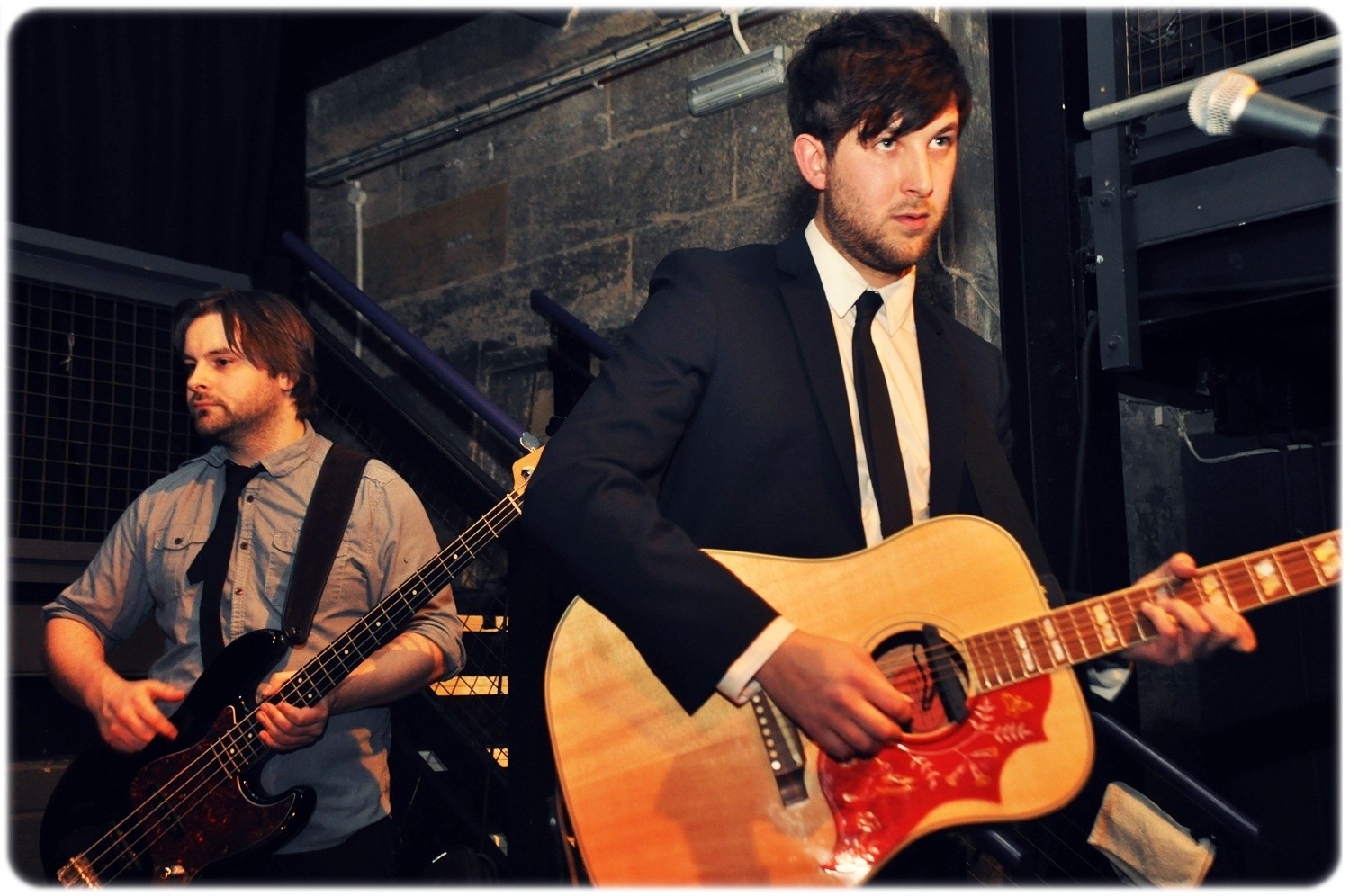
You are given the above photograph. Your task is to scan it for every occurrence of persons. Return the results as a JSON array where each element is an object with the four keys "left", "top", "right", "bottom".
[
  {"left": 517, "top": 7, "right": 1260, "bottom": 883},
  {"left": 37, "top": 284, "right": 467, "bottom": 877}
]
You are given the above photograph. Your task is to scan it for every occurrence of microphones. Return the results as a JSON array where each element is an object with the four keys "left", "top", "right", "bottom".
[{"left": 1188, "top": 71, "right": 1339, "bottom": 157}]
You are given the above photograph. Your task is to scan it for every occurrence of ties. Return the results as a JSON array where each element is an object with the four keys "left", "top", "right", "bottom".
[
  {"left": 186, "top": 461, "right": 268, "bottom": 670},
  {"left": 851, "top": 290, "right": 913, "bottom": 538}
]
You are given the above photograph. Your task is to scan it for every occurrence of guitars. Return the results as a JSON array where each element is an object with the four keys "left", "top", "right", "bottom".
[
  {"left": 541, "top": 513, "right": 1343, "bottom": 887},
  {"left": 37, "top": 443, "right": 548, "bottom": 888}
]
